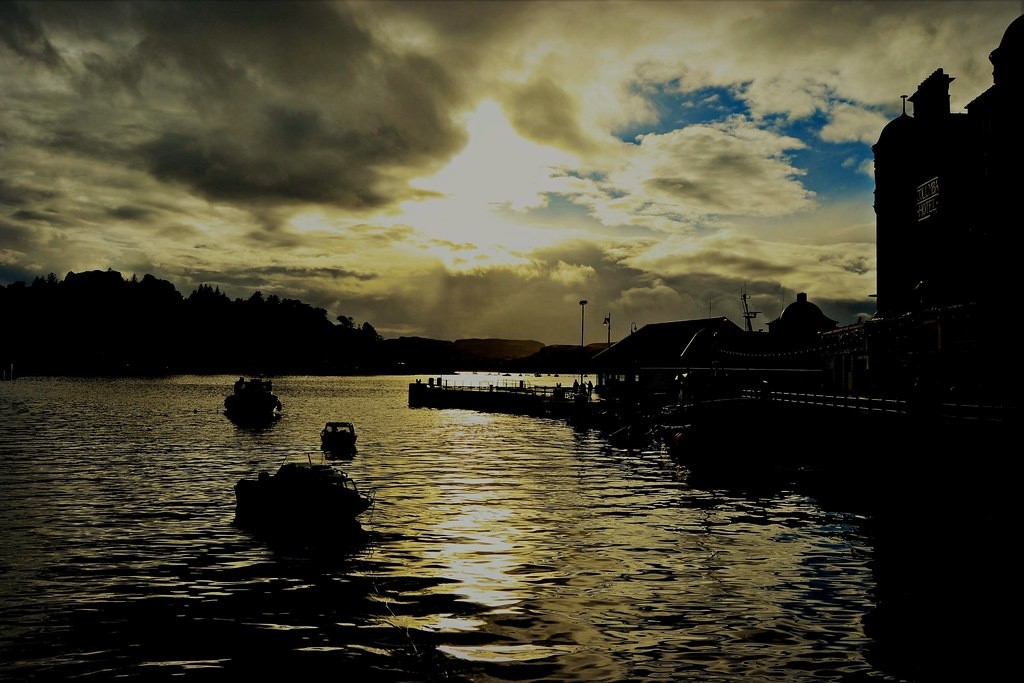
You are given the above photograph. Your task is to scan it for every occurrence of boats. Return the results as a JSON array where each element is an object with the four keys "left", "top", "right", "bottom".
[
  {"left": 316, "top": 421, "right": 360, "bottom": 453},
  {"left": 224, "top": 373, "right": 284, "bottom": 419},
  {"left": 224, "top": 450, "right": 378, "bottom": 564}
]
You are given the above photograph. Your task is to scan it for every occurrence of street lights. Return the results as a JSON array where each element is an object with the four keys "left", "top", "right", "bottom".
[
  {"left": 601, "top": 312, "right": 611, "bottom": 347},
  {"left": 631, "top": 321, "right": 638, "bottom": 335},
  {"left": 579, "top": 299, "right": 589, "bottom": 390}
]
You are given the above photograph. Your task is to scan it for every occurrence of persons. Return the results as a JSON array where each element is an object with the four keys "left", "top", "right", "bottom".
[{"left": 573, "top": 379, "right": 593, "bottom": 398}]
